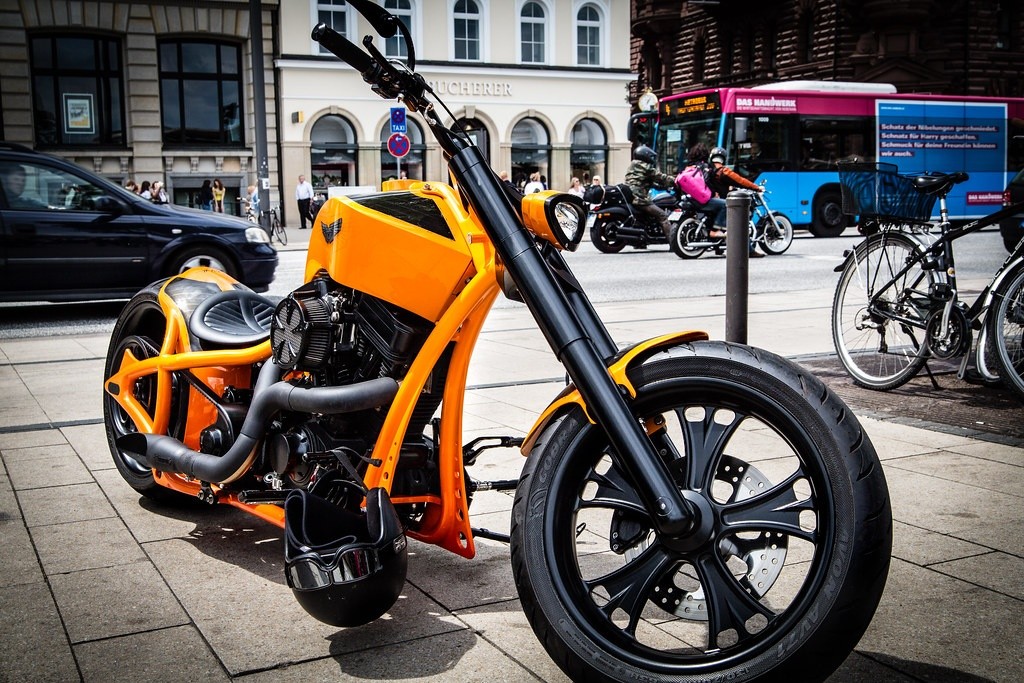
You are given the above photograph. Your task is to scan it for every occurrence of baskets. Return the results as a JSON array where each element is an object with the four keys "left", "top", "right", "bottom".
[{"left": 838, "top": 162, "right": 937, "bottom": 222}]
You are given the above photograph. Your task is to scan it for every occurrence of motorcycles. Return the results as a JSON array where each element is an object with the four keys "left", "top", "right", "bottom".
[
  {"left": 103, "top": 0, "right": 892, "bottom": 683},
  {"left": 582, "top": 174, "right": 706, "bottom": 254},
  {"left": 668, "top": 179, "right": 794, "bottom": 259}
]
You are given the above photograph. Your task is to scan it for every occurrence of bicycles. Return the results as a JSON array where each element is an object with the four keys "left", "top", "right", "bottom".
[
  {"left": 236, "top": 197, "right": 260, "bottom": 223},
  {"left": 829, "top": 164, "right": 1024, "bottom": 397},
  {"left": 262, "top": 205, "right": 288, "bottom": 247}
]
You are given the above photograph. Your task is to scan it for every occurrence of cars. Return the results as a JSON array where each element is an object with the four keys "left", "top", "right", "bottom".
[{"left": 0, "top": 139, "right": 279, "bottom": 304}]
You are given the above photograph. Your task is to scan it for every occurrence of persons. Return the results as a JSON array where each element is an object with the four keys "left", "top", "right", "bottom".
[
  {"left": 125, "top": 180, "right": 170, "bottom": 203},
  {"left": 295, "top": 175, "right": 314, "bottom": 229},
  {"left": 685, "top": 142, "right": 764, "bottom": 237},
  {"left": 247, "top": 184, "right": 261, "bottom": 222},
  {"left": 624, "top": 145, "right": 676, "bottom": 245},
  {"left": 499, "top": 170, "right": 547, "bottom": 195},
  {"left": 400, "top": 170, "right": 407, "bottom": 179},
  {"left": 1, "top": 165, "right": 59, "bottom": 210},
  {"left": 201, "top": 179, "right": 226, "bottom": 213},
  {"left": 567, "top": 173, "right": 601, "bottom": 201}
]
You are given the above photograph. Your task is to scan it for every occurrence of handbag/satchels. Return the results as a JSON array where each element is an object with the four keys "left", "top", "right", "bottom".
[{"left": 675, "top": 166, "right": 712, "bottom": 205}]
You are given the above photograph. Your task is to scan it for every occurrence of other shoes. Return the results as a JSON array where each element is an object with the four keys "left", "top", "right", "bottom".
[
  {"left": 750, "top": 252, "right": 764, "bottom": 257},
  {"left": 710, "top": 230, "right": 726, "bottom": 238},
  {"left": 311, "top": 219, "right": 314, "bottom": 229},
  {"left": 299, "top": 227, "right": 306, "bottom": 229},
  {"left": 715, "top": 250, "right": 724, "bottom": 255}
]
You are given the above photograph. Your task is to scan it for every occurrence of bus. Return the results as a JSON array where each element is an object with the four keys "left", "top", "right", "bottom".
[{"left": 626, "top": 80, "right": 1024, "bottom": 238}]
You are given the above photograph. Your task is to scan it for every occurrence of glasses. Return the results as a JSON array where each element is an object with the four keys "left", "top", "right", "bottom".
[{"left": 594, "top": 179, "right": 598, "bottom": 181}]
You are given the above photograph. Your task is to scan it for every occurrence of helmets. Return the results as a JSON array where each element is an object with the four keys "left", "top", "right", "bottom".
[
  {"left": 285, "top": 488, "right": 407, "bottom": 627},
  {"left": 633, "top": 146, "right": 657, "bottom": 164},
  {"left": 710, "top": 147, "right": 727, "bottom": 162}
]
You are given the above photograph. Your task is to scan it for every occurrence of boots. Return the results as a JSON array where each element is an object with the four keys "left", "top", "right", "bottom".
[
  {"left": 634, "top": 246, "right": 647, "bottom": 249},
  {"left": 660, "top": 220, "right": 674, "bottom": 252}
]
